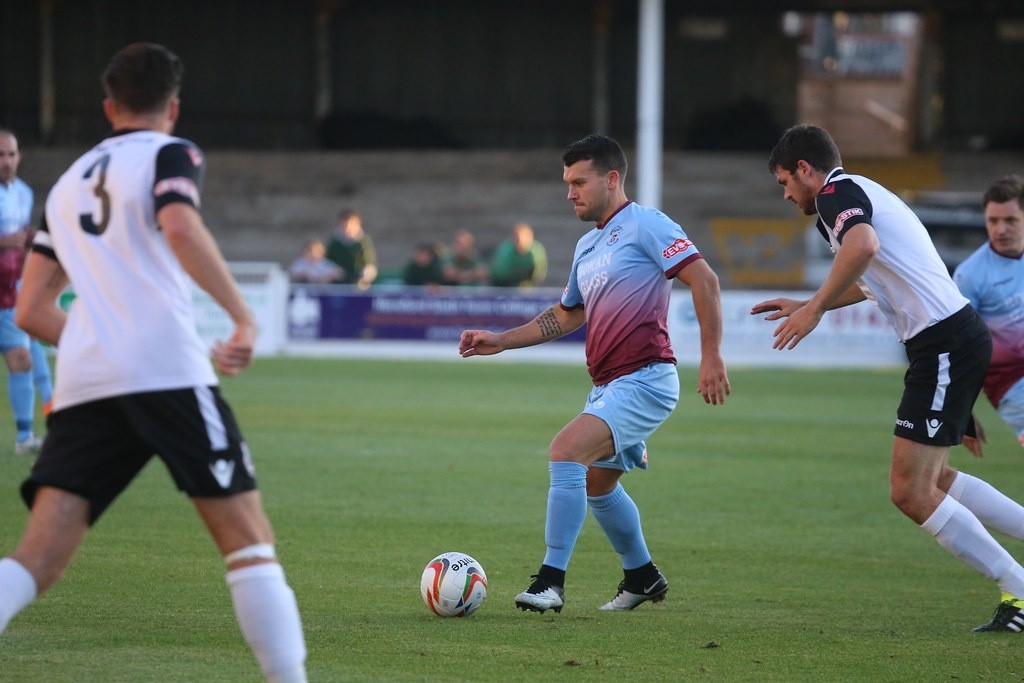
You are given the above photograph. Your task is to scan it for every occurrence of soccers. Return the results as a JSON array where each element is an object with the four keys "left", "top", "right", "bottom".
[{"left": 420, "top": 552, "right": 489, "bottom": 618}]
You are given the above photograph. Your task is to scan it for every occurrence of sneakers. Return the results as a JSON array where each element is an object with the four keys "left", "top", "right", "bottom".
[
  {"left": 515, "top": 577, "right": 565, "bottom": 615},
  {"left": 971, "top": 602, "right": 1024, "bottom": 633},
  {"left": 15, "top": 435, "right": 44, "bottom": 455},
  {"left": 598, "top": 572, "right": 668, "bottom": 610}
]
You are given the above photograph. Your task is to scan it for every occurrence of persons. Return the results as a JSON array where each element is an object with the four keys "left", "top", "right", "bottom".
[
  {"left": 952, "top": 172, "right": 1023, "bottom": 461},
  {"left": 437, "top": 228, "right": 490, "bottom": 288},
  {"left": 26, "top": 335, "right": 58, "bottom": 420},
  {"left": 487, "top": 222, "right": 548, "bottom": 288},
  {"left": 1, "top": 127, "right": 47, "bottom": 455},
  {"left": 0, "top": 42, "right": 310, "bottom": 681},
  {"left": 321, "top": 208, "right": 379, "bottom": 286},
  {"left": 285, "top": 235, "right": 346, "bottom": 285},
  {"left": 457, "top": 134, "right": 730, "bottom": 616},
  {"left": 399, "top": 239, "right": 447, "bottom": 287},
  {"left": 747, "top": 124, "right": 1023, "bottom": 636}
]
